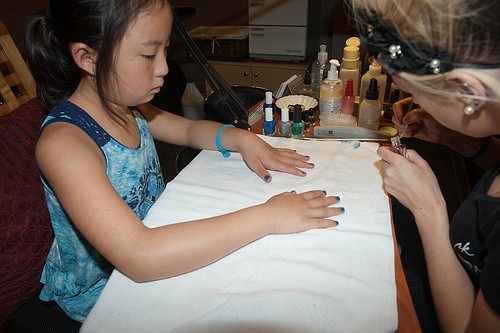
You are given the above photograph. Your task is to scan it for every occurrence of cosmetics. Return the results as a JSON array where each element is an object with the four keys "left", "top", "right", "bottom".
[{"left": 262, "top": 36, "right": 418, "bottom": 158}]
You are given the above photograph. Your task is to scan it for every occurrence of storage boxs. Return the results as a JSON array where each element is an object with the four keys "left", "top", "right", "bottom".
[
  {"left": 248, "top": 0, "right": 310, "bottom": 25},
  {"left": 188, "top": 26, "right": 249, "bottom": 61},
  {"left": 248, "top": 25, "right": 311, "bottom": 62}
]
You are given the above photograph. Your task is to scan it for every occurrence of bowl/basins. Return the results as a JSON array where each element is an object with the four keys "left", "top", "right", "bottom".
[{"left": 274, "top": 95, "right": 318, "bottom": 119}]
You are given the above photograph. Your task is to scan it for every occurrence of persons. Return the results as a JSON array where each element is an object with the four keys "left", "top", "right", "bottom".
[
  {"left": 26, "top": 1, "right": 347, "bottom": 321},
  {"left": 349, "top": 0, "right": 500, "bottom": 332}
]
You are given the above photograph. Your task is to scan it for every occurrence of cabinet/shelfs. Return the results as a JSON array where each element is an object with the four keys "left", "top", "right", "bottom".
[{"left": 204, "top": 63, "right": 308, "bottom": 101}]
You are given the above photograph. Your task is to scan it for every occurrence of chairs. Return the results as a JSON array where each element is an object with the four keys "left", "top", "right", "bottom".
[{"left": 0, "top": 22, "right": 101, "bottom": 333}]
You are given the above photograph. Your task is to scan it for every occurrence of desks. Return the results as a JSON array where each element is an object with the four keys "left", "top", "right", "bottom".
[{"left": 80, "top": 95, "right": 422, "bottom": 333}]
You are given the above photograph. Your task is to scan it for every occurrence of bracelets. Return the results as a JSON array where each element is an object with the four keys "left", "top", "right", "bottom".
[{"left": 215, "top": 123, "right": 235, "bottom": 158}]
